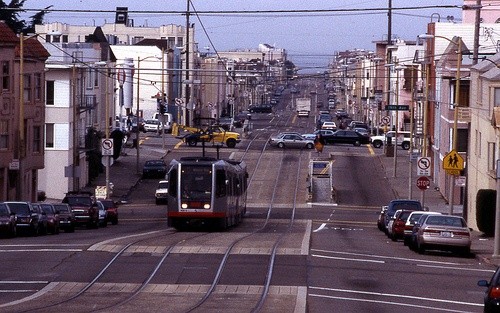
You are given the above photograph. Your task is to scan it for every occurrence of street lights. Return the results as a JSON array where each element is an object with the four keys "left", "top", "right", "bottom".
[
  {"left": 136, "top": 55, "right": 165, "bottom": 168},
  {"left": 72, "top": 61, "right": 107, "bottom": 191},
  {"left": 177, "top": 41, "right": 199, "bottom": 126},
  {"left": 417, "top": 34, "right": 463, "bottom": 217},
  {"left": 384, "top": 63, "right": 400, "bottom": 178},
  {"left": 107, "top": 63, "right": 136, "bottom": 197},
  {"left": 394, "top": 66, "right": 430, "bottom": 207},
  {"left": 14, "top": 30, "right": 64, "bottom": 200},
  {"left": 161, "top": 46, "right": 184, "bottom": 137}
]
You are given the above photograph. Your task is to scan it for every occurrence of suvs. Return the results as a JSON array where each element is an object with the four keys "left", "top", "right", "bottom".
[
  {"left": 371, "top": 130, "right": 416, "bottom": 150},
  {"left": 381, "top": 199, "right": 425, "bottom": 236},
  {"left": 62, "top": 190, "right": 100, "bottom": 230}
]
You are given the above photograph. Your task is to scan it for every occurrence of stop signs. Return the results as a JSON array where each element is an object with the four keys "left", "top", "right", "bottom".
[{"left": 417, "top": 176, "right": 430, "bottom": 191}]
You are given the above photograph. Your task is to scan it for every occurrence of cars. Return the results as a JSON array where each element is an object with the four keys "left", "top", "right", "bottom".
[
  {"left": 318, "top": 128, "right": 371, "bottom": 147},
  {"left": 114, "top": 78, "right": 372, "bottom": 150},
  {"left": 100, "top": 198, "right": 120, "bottom": 226},
  {"left": 376, "top": 205, "right": 445, "bottom": 252},
  {"left": 96, "top": 200, "right": 109, "bottom": 228},
  {"left": 142, "top": 159, "right": 167, "bottom": 178},
  {"left": 414, "top": 213, "right": 474, "bottom": 257},
  {"left": 477, "top": 266, "right": 500, "bottom": 313},
  {"left": 154, "top": 180, "right": 169, "bottom": 205},
  {"left": 0, "top": 190, "right": 76, "bottom": 238}
]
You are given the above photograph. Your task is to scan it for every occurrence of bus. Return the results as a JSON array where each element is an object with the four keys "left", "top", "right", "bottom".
[{"left": 165, "top": 117, "right": 249, "bottom": 232}]
